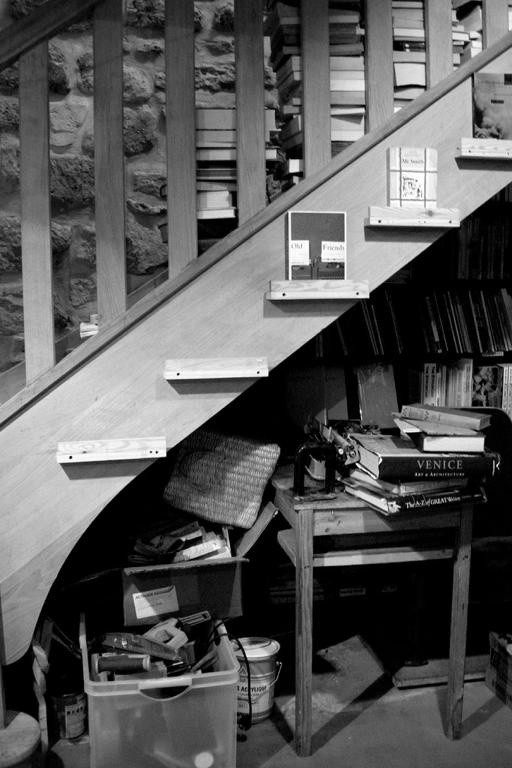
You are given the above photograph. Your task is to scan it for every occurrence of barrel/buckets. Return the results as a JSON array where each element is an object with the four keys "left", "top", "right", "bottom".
[
  {"left": 46, "top": 674, "right": 88, "bottom": 740},
  {"left": 229, "top": 637, "right": 283, "bottom": 724}
]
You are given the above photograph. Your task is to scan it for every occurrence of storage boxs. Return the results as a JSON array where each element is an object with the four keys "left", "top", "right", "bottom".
[
  {"left": 112, "top": 526, "right": 248, "bottom": 625},
  {"left": 75, "top": 611, "right": 238, "bottom": 766},
  {"left": 486, "top": 633, "right": 512, "bottom": 703}
]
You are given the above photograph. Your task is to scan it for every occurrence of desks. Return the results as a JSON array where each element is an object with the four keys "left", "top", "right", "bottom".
[{"left": 269, "top": 463, "right": 483, "bottom": 758}]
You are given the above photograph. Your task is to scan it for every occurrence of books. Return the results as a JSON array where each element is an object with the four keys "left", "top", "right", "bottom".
[
  {"left": 270, "top": 182, "right": 512, "bottom": 518},
  {"left": 196, "top": 0, "right": 483, "bottom": 253}
]
